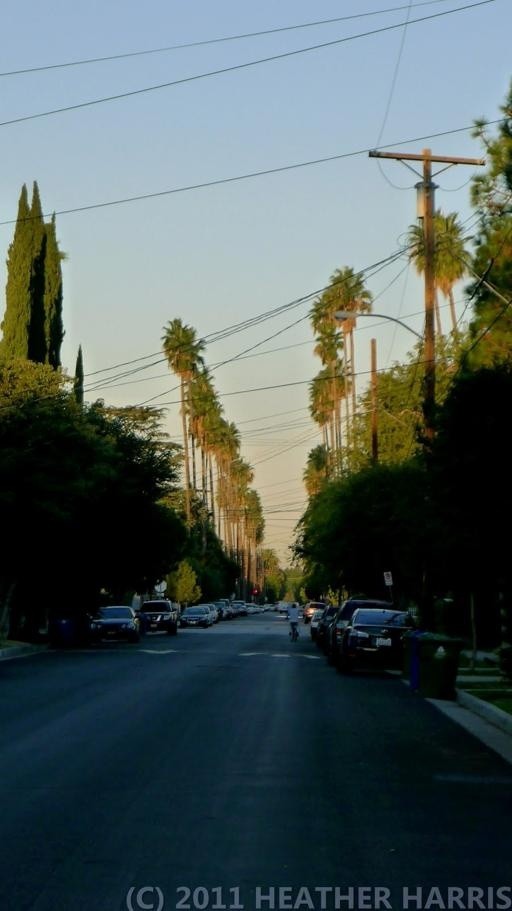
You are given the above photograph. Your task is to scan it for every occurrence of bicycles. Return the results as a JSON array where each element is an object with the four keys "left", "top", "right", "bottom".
[{"left": 284, "top": 618, "right": 300, "bottom": 642}]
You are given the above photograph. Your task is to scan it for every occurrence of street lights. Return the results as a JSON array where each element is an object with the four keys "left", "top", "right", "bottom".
[{"left": 331, "top": 150, "right": 487, "bottom": 631}]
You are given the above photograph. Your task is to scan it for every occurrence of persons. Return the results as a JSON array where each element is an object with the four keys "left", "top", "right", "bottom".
[{"left": 287, "top": 603, "right": 299, "bottom": 630}]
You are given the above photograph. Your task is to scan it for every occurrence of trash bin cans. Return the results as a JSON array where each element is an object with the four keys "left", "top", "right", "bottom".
[{"left": 401, "top": 637, "right": 464, "bottom": 701}]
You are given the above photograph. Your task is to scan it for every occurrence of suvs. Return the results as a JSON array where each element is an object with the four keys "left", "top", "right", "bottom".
[{"left": 135, "top": 599, "right": 178, "bottom": 634}]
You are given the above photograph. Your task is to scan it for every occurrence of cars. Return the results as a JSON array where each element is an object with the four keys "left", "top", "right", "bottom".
[
  {"left": 179, "top": 599, "right": 299, "bottom": 628},
  {"left": 86, "top": 605, "right": 141, "bottom": 644},
  {"left": 303, "top": 598, "right": 417, "bottom": 676}
]
